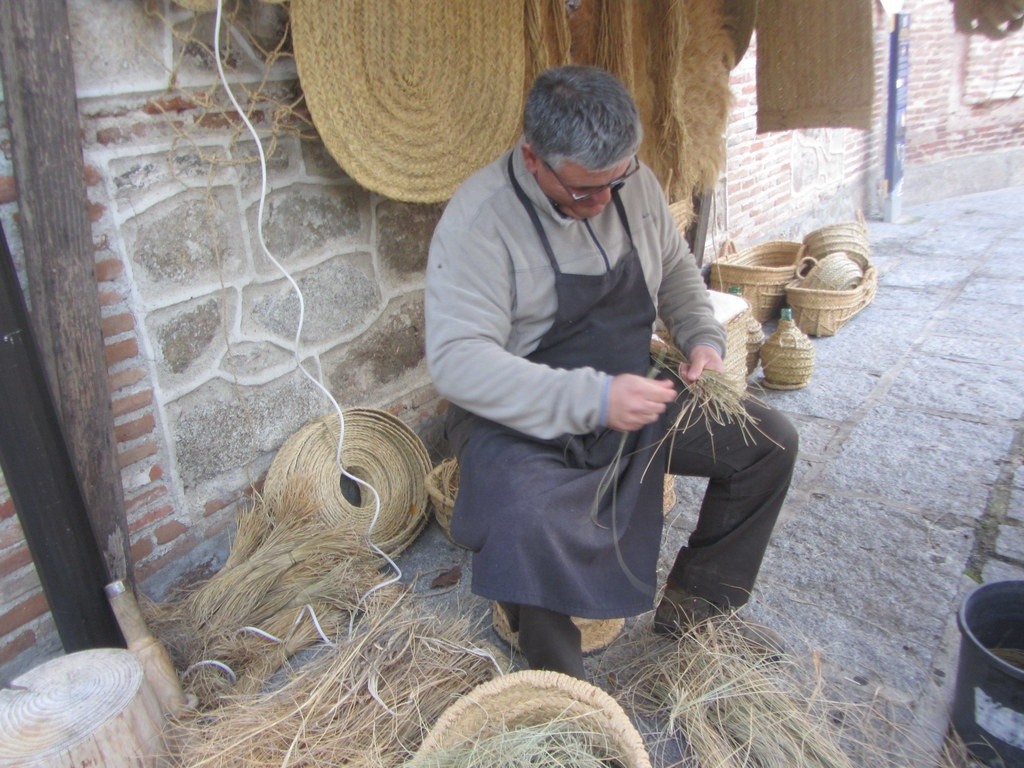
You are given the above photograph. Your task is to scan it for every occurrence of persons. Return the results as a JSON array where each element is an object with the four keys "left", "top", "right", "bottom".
[{"left": 421, "top": 62, "right": 799, "bottom": 688}]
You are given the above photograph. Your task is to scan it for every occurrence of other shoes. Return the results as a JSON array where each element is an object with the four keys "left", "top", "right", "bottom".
[{"left": 653, "top": 588, "right": 785, "bottom": 668}]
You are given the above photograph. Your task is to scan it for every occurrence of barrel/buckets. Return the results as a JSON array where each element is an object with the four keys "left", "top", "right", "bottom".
[{"left": 953, "top": 579, "right": 1024, "bottom": 767}]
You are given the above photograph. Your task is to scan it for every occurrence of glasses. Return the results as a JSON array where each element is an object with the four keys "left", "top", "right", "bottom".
[{"left": 540, "top": 154, "right": 640, "bottom": 203}]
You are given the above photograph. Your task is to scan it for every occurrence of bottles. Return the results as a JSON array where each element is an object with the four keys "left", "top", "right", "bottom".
[
  {"left": 760, "top": 308, "right": 815, "bottom": 390},
  {"left": 725, "top": 287, "right": 765, "bottom": 376}
]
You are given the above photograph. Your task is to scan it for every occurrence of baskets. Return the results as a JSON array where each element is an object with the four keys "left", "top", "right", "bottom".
[
  {"left": 785, "top": 267, "right": 877, "bottom": 336},
  {"left": 413, "top": 668, "right": 651, "bottom": 768},
  {"left": 796, "top": 251, "right": 862, "bottom": 291},
  {"left": 798, "top": 209, "right": 869, "bottom": 260},
  {"left": 710, "top": 240, "right": 802, "bottom": 322},
  {"left": 424, "top": 458, "right": 461, "bottom": 530}
]
